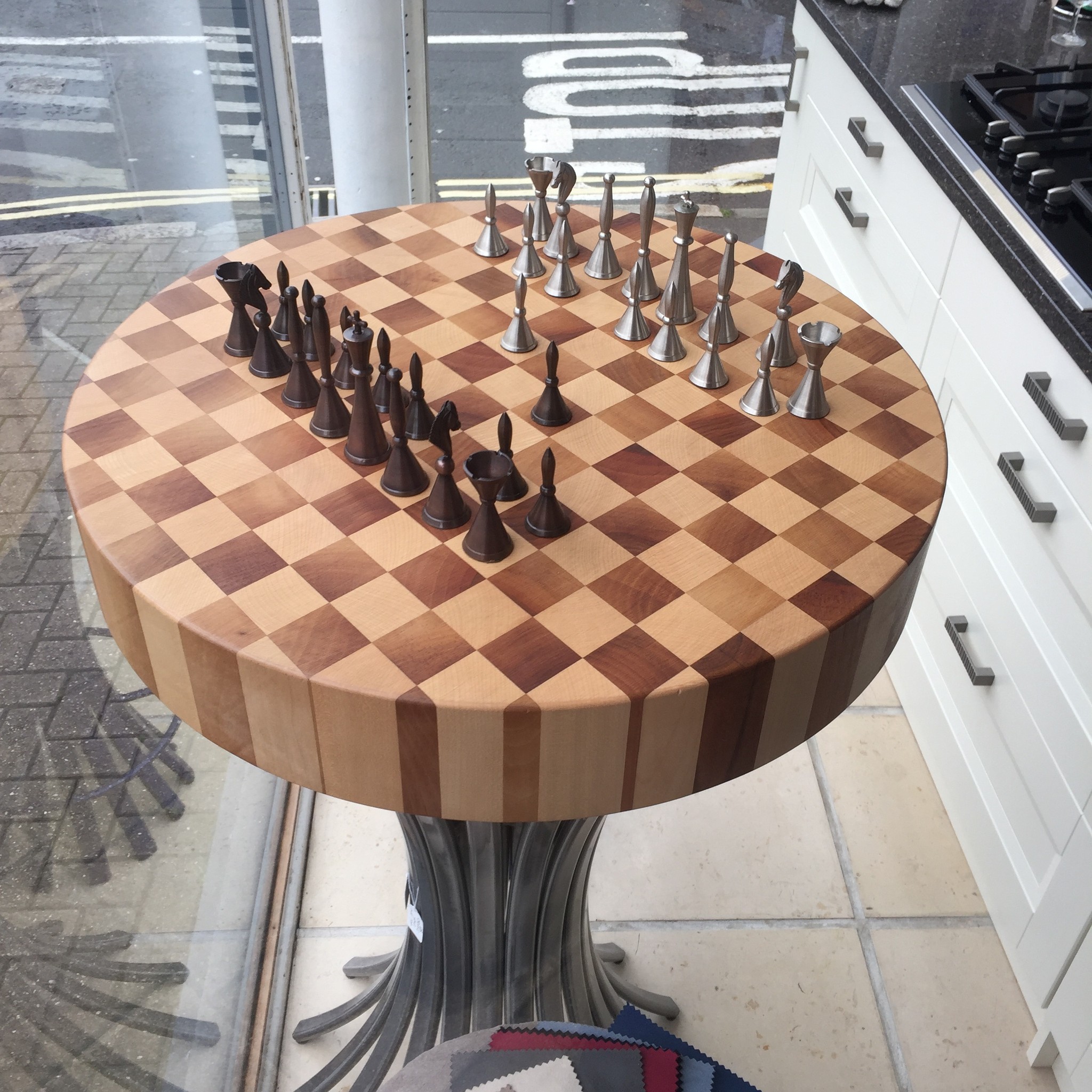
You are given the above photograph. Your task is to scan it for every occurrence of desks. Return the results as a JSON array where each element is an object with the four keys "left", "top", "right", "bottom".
[
  {"left": 60, "top": 196, "right": 949, "bottom": 1092},
  {"left": 377, "top": 1002, "right": 762, "bottom": 1092}
]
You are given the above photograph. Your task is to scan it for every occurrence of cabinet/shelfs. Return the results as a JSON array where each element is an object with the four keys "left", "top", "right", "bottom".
[
  {"left": 882, "top": 215, "right": 1092, "bottom": 1028},
  {"left": 760, "top": 0, "right": 959, "bottom": 370}
]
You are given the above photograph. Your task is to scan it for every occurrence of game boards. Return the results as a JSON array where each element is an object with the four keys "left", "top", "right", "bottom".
[{"left": 62, "top": 199, "right": 952, "bottom": 707}]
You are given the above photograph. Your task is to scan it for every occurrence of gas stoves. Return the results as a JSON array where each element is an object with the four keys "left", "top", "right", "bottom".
[{"left": 900, "top": 54, "right": 1092, "bottom": 311}]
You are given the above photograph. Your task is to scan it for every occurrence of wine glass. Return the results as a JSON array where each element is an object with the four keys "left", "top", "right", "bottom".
[{"left": 1051, "top": 0, "right": 1092, "bottom": 48}]
